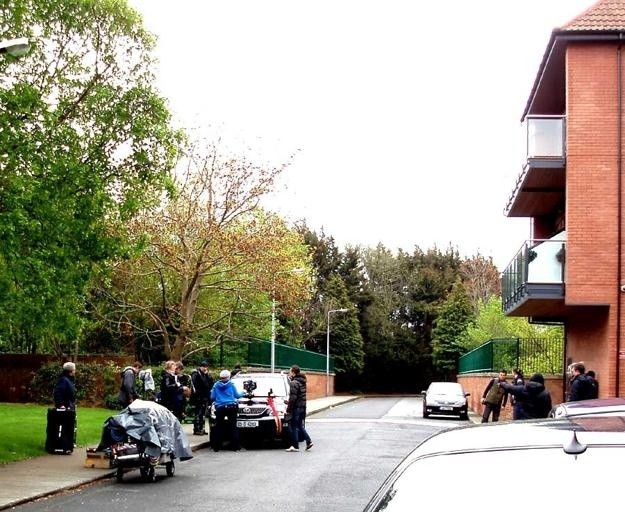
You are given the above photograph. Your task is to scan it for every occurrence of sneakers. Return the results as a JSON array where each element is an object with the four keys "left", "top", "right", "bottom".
[
  {"left": 286, "top": 445, "right": 299, "bottom": 452},
  {"left": 306, "top": 443, "right": 313, "bottom": 449}
]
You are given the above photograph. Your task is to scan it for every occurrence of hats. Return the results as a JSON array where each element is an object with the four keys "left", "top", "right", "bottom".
[{"left": 199, "top": 360, "right": 208, "bottom": 367}]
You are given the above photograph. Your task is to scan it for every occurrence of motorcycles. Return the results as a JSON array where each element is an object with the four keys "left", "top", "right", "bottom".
[{"left": 110, "top": 399, "right": 193, "bottom": 484}]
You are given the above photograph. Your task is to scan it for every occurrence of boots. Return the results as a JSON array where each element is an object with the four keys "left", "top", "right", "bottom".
[{"left": 193, "top": 420, "right": 209, "bottom": 435}]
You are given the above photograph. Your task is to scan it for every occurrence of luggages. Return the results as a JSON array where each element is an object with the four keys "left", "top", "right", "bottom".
[{"left": 45, "top": 408, "right": 75, "bottom": 455}]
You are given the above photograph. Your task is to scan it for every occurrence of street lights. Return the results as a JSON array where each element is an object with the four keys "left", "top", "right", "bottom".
[
  {"left": 326, "top": 307, "right": 348, "bottom": 396},
  {"left": 271, "top": 268, "right": 304, "bottom": 372}
]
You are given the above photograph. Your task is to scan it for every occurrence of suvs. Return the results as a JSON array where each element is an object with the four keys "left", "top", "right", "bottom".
[
  {"left": 206, "top": 368, "right": 305, "bottom": 447},
  {"left": 363, "top": 416, "right": 625, "bottom": 512}
]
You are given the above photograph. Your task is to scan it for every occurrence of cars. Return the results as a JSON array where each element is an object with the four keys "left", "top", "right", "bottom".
[
  {"left": 421, "top": 381, "right": 471, "bottom": 419},
  {"left": 549, "top": 396, "right": 625, "bottom": 418}
]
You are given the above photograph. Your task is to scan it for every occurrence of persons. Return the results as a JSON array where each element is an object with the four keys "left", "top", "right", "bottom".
[
  {"left": 189, "top": 360, "right": 215, "bottom": 435},
  {"left": 209, "top": 369, "right": 247, "bottom": 452},
  {"left": 159, "top": 360, "right": 188, "bottom": 418},
  {"left": 479, "top": 363, "right": 599, "bottom": 423},
  {"left": 117, "top": 361, "right": 143, "bottom": 407},
  {"left": 173, "top": 361, "right": 192, "bottom": 422},
  {"left": 283, "top": 365, "right": 313, "bottom": 452},
  {"left": 51, "top": 360, "right": 77, "bottom": 448}
]
